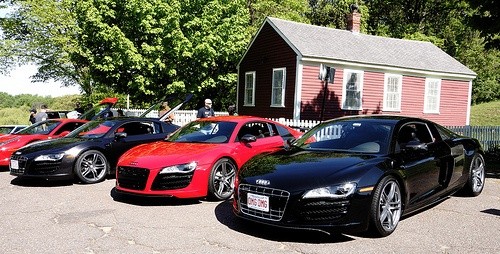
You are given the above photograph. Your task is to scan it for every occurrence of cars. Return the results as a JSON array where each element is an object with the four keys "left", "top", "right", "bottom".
[
  {"left": 115, "top": 116, "right": 317, "bottom": 202},
  {"left": 0, "top": 125, "right": 30, "bottom": 136},
  {"left": 0, "top": 117, "right": 124, "bottom": 171},
  {"left": 234, "top": 114, "right": 486, "bottom": 238},
  {"left": 8, "top": 115, "right": 182, "bottom": 184}
]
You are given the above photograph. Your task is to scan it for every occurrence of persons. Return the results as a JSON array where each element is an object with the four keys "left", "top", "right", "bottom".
[
  {"left": 227, "top": 102, "right": 239, "bottom": 116},
  {"left": 158, "top": 101, "right": 175, "bottom": 123},
  {"left": 33, "top": 104, "right": 49, "bottom": 122},
  {"left": 197, "top": 99, "right": 216, "bottom": 118},
  {"left": 74, "top": 102, "right": 125, "bottom": 120},
  {"left": 29, "top": 112, "right": 36, "bottom": 124}
]
